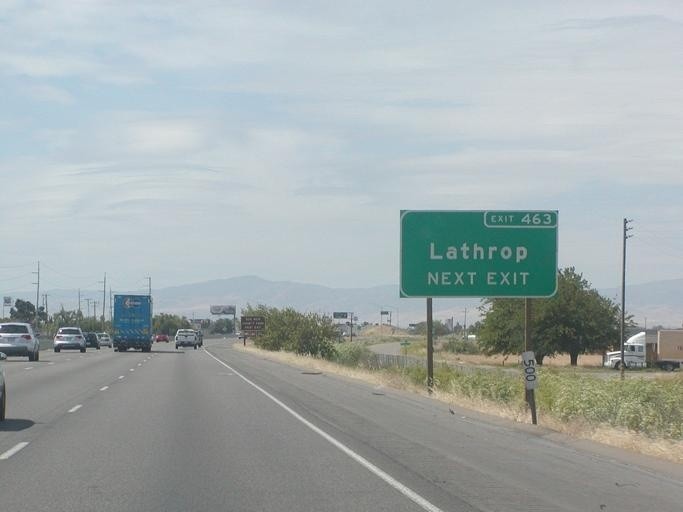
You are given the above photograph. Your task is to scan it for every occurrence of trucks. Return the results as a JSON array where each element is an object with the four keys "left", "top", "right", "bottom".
[
  {"left": 604, "top": 331, "right": 683, "bottom": 371},
  {"left": 113, "top": 294, "right": 153, "bottom": 351}
]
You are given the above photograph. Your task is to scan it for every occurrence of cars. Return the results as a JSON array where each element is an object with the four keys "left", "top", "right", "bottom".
[
  {"left": 0, "top": 352, "right": 7, "bottom": 421},
  {"left": 175, "top": 328, "right": 203, "bottom": 349},
  {"left": 53, "top": 327, "right": 112, "bottom": 353},
  {"left": 156, "top": 331, "right": 169, "bottom": 341}
]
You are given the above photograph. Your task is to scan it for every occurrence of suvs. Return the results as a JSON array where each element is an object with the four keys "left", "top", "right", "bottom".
[{"left": 0, "top": 323, "right": 40, "bottom": 362}]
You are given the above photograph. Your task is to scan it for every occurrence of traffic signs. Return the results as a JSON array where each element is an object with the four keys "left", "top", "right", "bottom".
[
  {"left": 399, "top": 209, "right": 559, "bottom": 300},
  {"left": 333, "top": 311, "right": 347, "bottom": 319}
]
